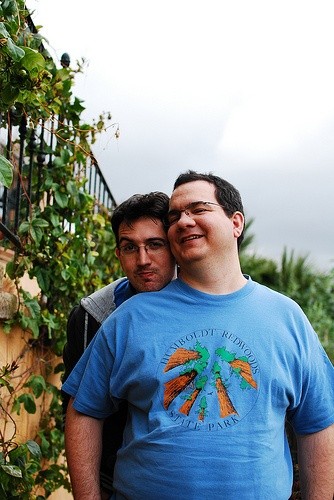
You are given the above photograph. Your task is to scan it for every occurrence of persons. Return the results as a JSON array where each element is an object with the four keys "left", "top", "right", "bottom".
[
  {"left": 60, "top": 171, "right": 334, "bottom": 500},
  {"left": 61, "top": 192, "right": 179, "bottom": 500}
]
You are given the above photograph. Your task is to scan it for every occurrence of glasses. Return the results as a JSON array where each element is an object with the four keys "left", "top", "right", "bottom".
[
  {"left": 118, "top": 241, "right": 170, "bottom": 255},
  {"left": 162, "top": 200, "right": 235, "bottom": 227}
]
